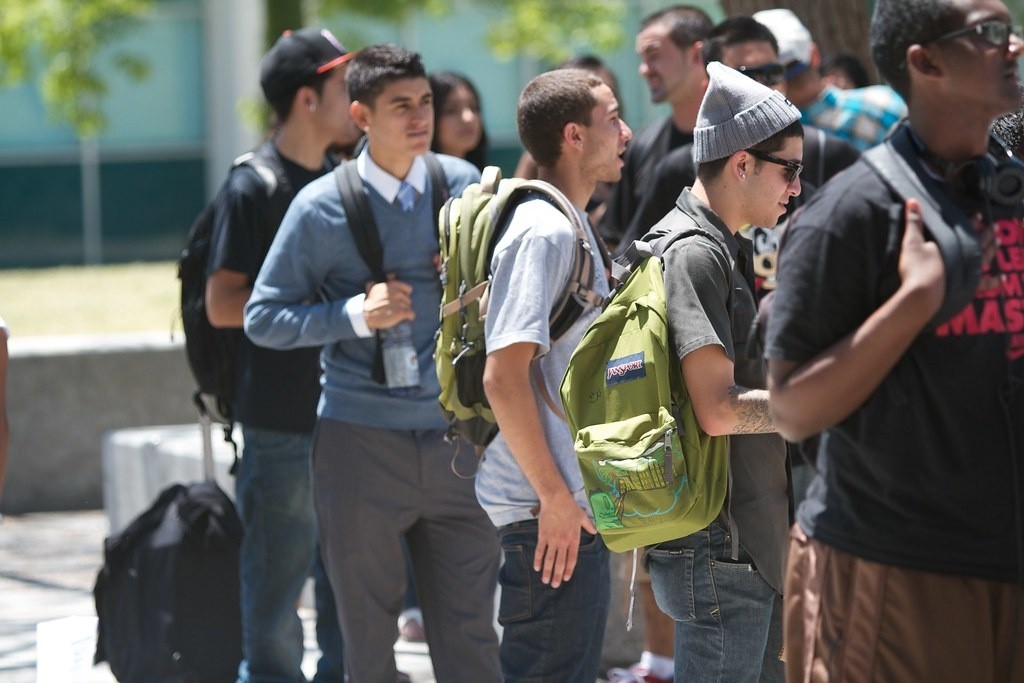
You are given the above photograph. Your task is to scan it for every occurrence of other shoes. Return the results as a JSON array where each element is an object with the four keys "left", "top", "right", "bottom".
[{"left": 394, "top": 606, "right": 429, "bottom": 655}]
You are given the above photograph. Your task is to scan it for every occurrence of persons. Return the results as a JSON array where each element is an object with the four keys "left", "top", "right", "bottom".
[
  {"left": 476, "top": 68, "right": 633, "bottom": 682},
  {"left": 399, "top": 5, "right": 911, "bottom": 683},
  {"left": 641, "top": 61, "right": 805, "bottom": 683},
  {"left": 765, "top": 0, "right": 1024, "bottom": 683},
  {"left": 202, "top": 27, "right": 367, "bottom": 683},
  {"left": 244, "top": 43, "right": 504, "bottom": 682}
]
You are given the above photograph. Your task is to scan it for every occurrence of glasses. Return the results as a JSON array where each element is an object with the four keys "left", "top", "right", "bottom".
[
  {"left": 745, "top": 148, "right": 805, "bottom": 183},
  {"left": 898, "top": 20, "right": 1020, "bottom": 67},
  {"left": 733, "top": 64, "right": 787, "bottom": 86}
]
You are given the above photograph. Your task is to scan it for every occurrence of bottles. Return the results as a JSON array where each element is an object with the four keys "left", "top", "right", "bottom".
[{"left": 380, "top": 322, "right": 421, "bottom": 388}]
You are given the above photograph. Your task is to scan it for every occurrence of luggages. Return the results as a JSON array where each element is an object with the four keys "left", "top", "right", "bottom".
[{"left": 93, "top": 391, "right": 247, "bottom": 683}]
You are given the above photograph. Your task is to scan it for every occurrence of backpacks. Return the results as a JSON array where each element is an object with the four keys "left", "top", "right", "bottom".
[
  {"left": 558, "top": 228, "right": 738, "bottom": 631},
  {"left": 432, "top": 166, "right": 622, "bottom": 480},
  {"left": 175, "top": 151, "right": 278, "bottom": 474}
]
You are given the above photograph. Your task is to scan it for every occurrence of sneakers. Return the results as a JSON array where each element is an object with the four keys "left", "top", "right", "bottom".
[{"left": 607, "top": 662, "right": 674, "bottom": 683}]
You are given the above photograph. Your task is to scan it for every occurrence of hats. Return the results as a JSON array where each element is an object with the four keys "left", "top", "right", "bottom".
[
  {"left": 752, "top": 9, "right": 813, "bottom": 77},
  {"left": 261, "top": 27, "right": 358, "bottom": 105},
  {"left": 693, "top": 61, "right": 803, "bottom": 163}
]
